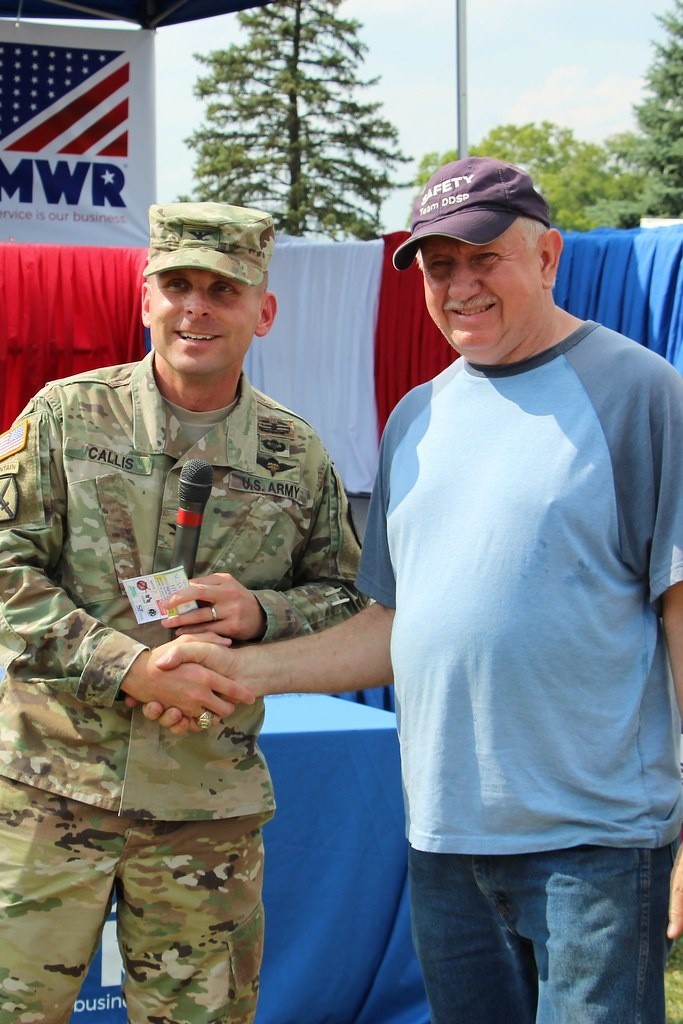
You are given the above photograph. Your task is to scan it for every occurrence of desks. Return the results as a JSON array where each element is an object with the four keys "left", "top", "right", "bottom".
[{"left": 63, "top": 694, "right": 439, "bottom": 1024}]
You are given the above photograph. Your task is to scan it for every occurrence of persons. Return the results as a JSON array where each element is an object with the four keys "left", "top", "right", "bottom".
[
  {"left": 124, "top": 158, "right": 683, "bottom": 1024},
  {"left": 1, "top": 200, "right": 368, "bottom": 1023}
]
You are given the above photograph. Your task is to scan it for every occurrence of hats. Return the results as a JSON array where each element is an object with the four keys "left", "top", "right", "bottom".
[
  {"left": 142, "top": 201, "right": 275, "bottom": 286},
  {"left": 392, "top": 157, "right": 551, "bottom": 271}
]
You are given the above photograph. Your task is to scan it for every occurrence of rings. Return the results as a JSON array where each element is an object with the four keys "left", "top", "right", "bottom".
[
  {"left": 198, "top": 712, "right": 213, "bottom": 729},
  {"left": 211, "top": 605, "right": 217, "bottom": 620}
]
